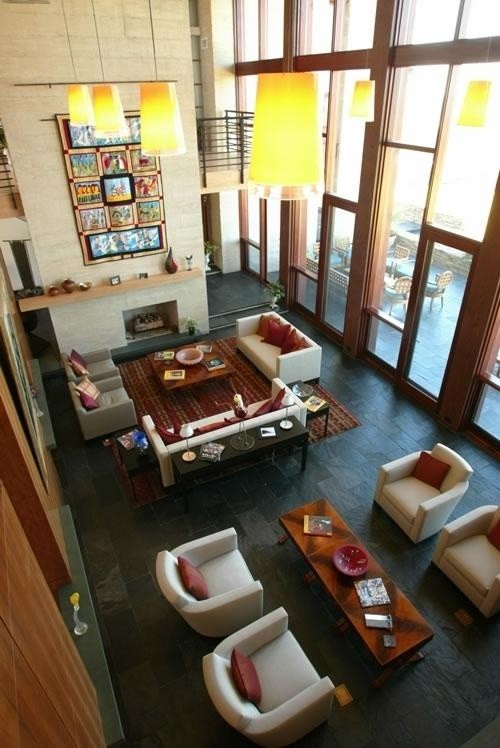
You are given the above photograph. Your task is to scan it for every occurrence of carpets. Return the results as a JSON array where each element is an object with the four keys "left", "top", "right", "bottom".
[{"left": 110, "top": 337, "right": 361, "bottom": 510}]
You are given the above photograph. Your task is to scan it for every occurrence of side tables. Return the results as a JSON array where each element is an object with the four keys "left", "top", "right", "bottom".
[
  {"left": 113, "top": 429, "right": 158, "bottom": 502},
  {"left": 285, "top": 380, "right": 330, "bottom": 437}
]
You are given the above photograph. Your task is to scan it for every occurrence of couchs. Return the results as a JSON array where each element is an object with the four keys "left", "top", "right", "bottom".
[
  {"left": 142, "top": 378, "right": 308, "bottom": 489},
  {"left": 235, "top": 311, "right": 322, "bottom": 389}
]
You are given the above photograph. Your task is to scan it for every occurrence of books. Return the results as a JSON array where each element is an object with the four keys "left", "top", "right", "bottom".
[
  {"left": 164, "top": 369, "right": 185, "bottom": 380},
  {"left": 154, "top": 351, "right": 175, "bottom": 361},
  {"left": 198, "top": 441, "right": 226, "bottom": 462},
  {"left": 196, "top": 344, "right": 213, "bottom": 352},
  {"left": 204, "top": 358, "right": 226, "bottom": 371},
  {"left": 302, "top": 514, "right": 333, "bottom": 537}
]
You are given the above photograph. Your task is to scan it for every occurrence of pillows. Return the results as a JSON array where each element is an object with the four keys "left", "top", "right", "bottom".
[
  {"left": 155, "top": 388, "right": 289, "bottom": 444},
  {"left": 75, "top": 377, "right": 100, "bottom": 409},
  {"left": 257, "top": 315, "right": 309, "bottom": 355},
  {"left": 68, "top": 349, "right": 90, "bottom": 376}
]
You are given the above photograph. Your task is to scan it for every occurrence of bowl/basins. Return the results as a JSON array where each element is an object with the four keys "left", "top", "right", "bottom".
[
  {"left": 292, "top": 382, "right": 314, "bottom": 399},
  {"left": 175, "top": 347, "right": 204, "bottom": 366},
  {"left": 332, "top": 544, "right": 370, "bottom": 576}
]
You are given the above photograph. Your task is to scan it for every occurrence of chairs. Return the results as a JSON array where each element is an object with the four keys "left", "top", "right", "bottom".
[
  {"left": 156, "top": 528, "right": 335, "bottom": 748},
  {"left": 67, "top": 376, "right": 138, "bottom": 444},
  {"left": 374, "top": 442, "right": 499, "bottom": 619},
  {"left": 59, "top": 347, "right": 121, "bottom": 384},
  {"left": 313, "top": 235, "right": 454, "bottom": 316}
]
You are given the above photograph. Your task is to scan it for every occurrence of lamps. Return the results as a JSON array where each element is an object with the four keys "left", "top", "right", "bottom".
[
  {"left": 249, "top": 2, "right": 322, "bottom": 188},
  {"left": 350, "top": 0, "right": 377, "bottom": 121},
  {"left": 63, "top": 2, "right": 95, "bottom": 129},
  {"left": 455, "top": 1, "right": 499, "bottom": 129},
  {"left": 139, "top": 2, "right": 188, "bottom": 158},
  {"left": 90, "top": 0, "right": 131, "bottom": 139}
]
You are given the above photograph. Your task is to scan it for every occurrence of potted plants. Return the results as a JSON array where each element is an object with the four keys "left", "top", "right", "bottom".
[
  {"left": 262, "top": 281, "right": 284, "bottom": 309},
  {"left": 183, "top": 317, "right": 198, "bottom": 335},
  {"left": 204, "top": 241, "right": 218, "bottom": 270}
]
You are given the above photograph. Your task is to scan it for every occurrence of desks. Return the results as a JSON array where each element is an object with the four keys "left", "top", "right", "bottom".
[{"left": 170, "top": 416, "right": 309, "bottom": 513}]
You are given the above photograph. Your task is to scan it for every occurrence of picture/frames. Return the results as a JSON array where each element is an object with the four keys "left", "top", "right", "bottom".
[{"left": 110, "top": 276, "right": 121, "bottom": 286}]
